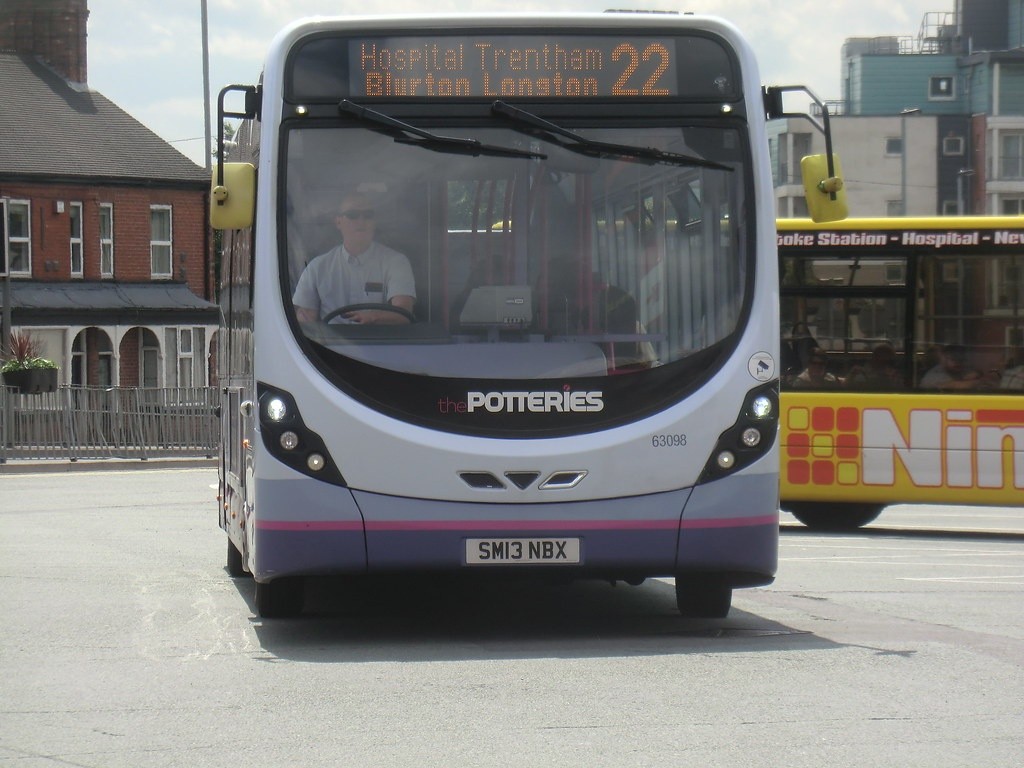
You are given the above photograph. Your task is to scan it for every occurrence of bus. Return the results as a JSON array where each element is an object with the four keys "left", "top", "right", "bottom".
[
  {"left": 203, "top": 14, "right": 850, "bottom": 627},
  {"left": 489, "top": 214, "right": 1023, "bottom": 530}
]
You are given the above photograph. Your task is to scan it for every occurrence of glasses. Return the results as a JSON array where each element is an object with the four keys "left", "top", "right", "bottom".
[{"left": 339, "top": 209, "right": 375, "bottom": 220}]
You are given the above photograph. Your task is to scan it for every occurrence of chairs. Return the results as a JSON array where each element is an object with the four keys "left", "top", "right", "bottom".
[
  {"left": 296, "top": 205, "right": 442, "bottom": 323},
  {"left": 840, "top": 366, "right": 879, "bottom": 391}
]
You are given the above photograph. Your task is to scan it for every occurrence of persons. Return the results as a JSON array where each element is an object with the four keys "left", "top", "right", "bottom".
[
  {"left": 293, "top": 192, "right": 416, "bottom": 323},
  {"left": 794, "top": 342, "right": 1024, "bottom": 390},
  {"left": 570, "top": 268, "right": 658, "bottom": 369}
]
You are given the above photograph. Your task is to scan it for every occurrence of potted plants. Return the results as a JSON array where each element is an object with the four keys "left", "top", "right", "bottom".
[{"left": 0, "top": 328, "right": 59, "bottom": 394}]
